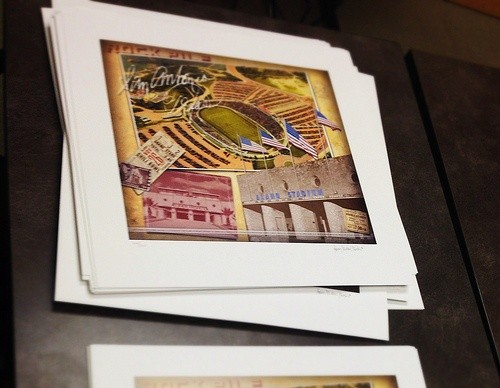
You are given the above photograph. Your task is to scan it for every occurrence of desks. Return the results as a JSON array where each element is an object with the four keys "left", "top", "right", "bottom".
[
  {"left": 2, "top": 0, "right": 499, "bottom": 388},
  {"left": 408, "top": 47, "right": 500, "bottom": 351}
]
